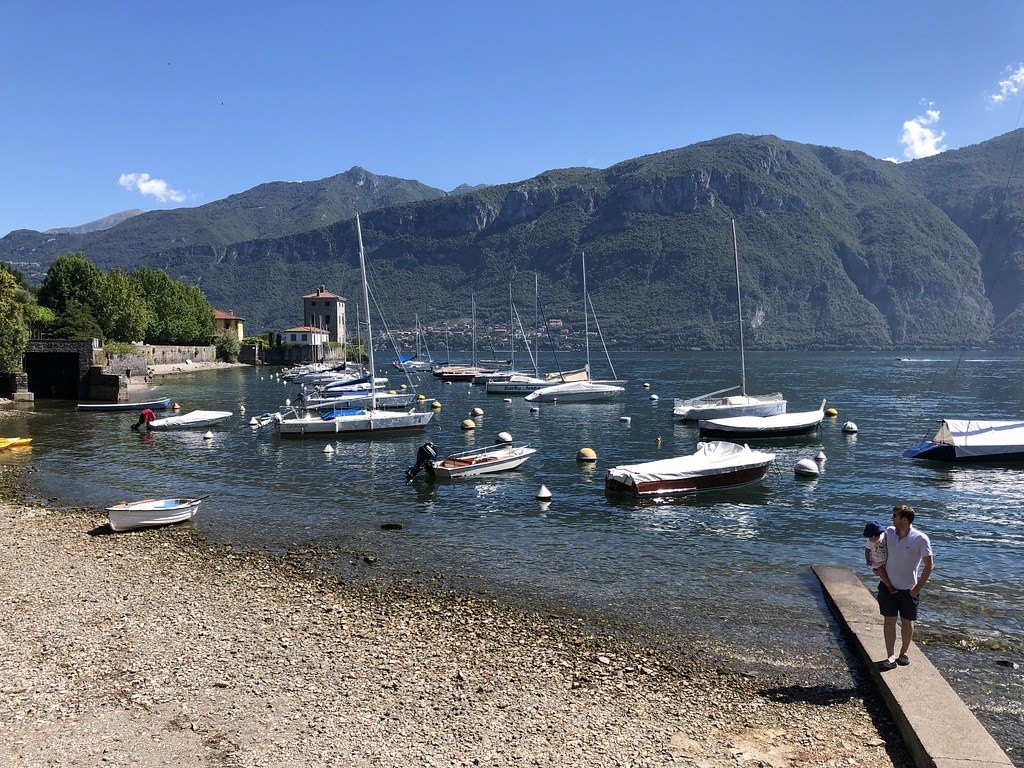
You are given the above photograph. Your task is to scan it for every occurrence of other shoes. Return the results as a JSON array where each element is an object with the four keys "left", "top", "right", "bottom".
[
  {"left": 877, "top": 659, "right": 897, "bottom": 669},
  {"left": 897, "top": 653, "right": 910, "bottom": 666}
]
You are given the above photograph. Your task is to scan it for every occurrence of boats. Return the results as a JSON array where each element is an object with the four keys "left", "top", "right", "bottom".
[
  {"left": 478, "top": 359, "right": 513, "bottom": 370},
  {"left": 606, "top": 440, "right": 775, "bottom": 496},
  {"left": 0, "top": 437, "right": 33, "bottom": 451},
  {"left": 699, "top": 398, "right": 827, "bottom": 444},
  {"left": 130, "top": 409, "right": 234, "bottom": 431},
  {"left": 105, "top": 498, "right": 202, "bottom": 531},
  {"left": 77, "top": 398, "right": 171, "bottom": 411},
  {"left": 896, "top": 356, "right": 910, "bottom": 361},
  {"left": 404, "top": 442, "right": 537, "bottom": 486}
]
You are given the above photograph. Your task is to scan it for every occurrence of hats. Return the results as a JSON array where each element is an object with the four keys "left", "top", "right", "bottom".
[{"left": 862, "top": 521, "right": 885, "bottom": 538}]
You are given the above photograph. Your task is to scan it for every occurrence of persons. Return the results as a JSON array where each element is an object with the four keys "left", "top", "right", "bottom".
[
  {"left": 863, "top": 521, "right": 916, "bottom": 594},
  {"left": 873, "top": 505, "right": 934, "bottom": 670}
]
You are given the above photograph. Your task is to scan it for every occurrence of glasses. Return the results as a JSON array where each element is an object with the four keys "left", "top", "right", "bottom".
[{"left": 892, "top": 514, "right": 902, "bottom": 518}]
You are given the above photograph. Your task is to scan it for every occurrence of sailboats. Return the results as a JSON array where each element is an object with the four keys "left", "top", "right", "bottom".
[
  {"left": 671, "top": 219, "right": 788, "bottom": 420},
  {"left": 525, "top": 252, "right": 625, "bottom": 402},
  {"left": 392, "top": 313, "right": 434, "bottom": 371},
  {"left": 902, "top": 104, "right": 1024, "bottom": 461},
  {"left": 272, "top": 212, "right": 434, "bottom": 433},
  {"left": 486, "top": 275, "right": 628, "bottom": 394},
  {"left": 433, "top": 293, "right": 499, "bottom": 381},
  {"left": 471, "top": 282, "right": 540, "bottom": 384}
]
